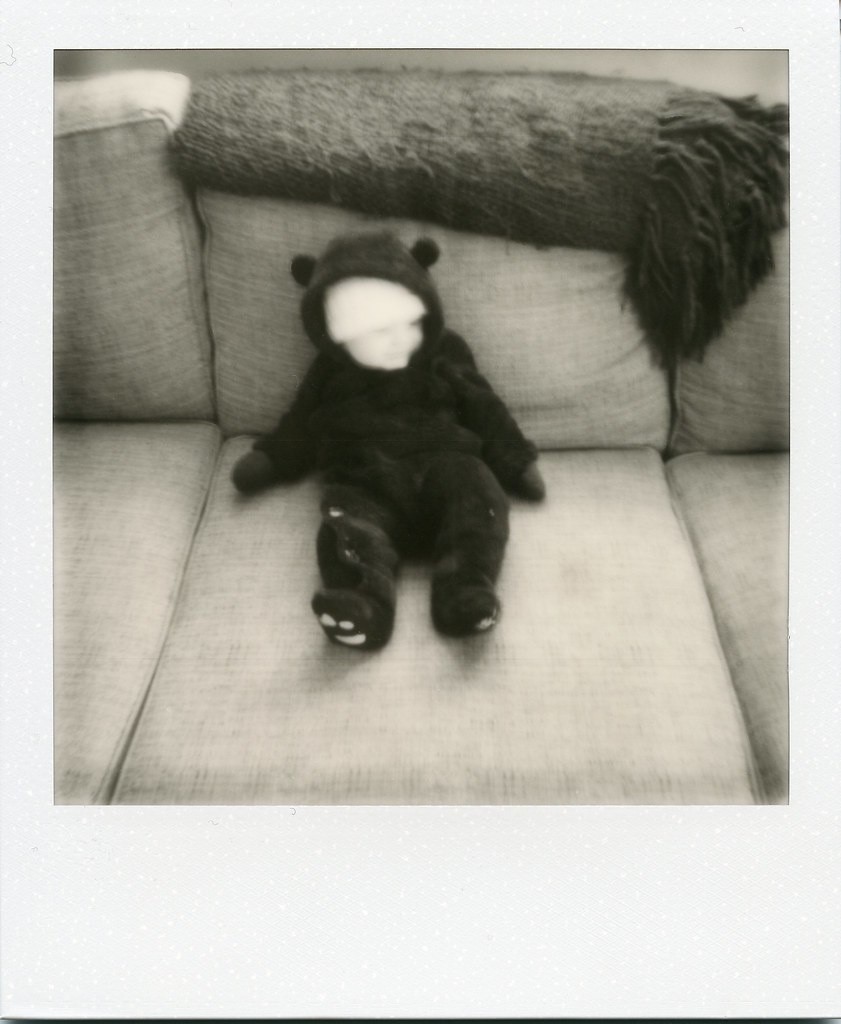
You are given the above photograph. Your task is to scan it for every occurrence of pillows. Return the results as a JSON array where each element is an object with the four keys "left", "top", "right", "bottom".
[
  {"left": 667, "top": 204, "right": 789, "bottom": 462},
  {"left": 55, "top": 69, "right": 220, "bottom": 424},
  {"left": 195, "top": 179, "right": 673, "bottom": 454}
]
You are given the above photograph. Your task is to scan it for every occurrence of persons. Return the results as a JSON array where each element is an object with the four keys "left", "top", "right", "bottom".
[{"left": 233, "top": 229, "right": 545, "bottom": 649}]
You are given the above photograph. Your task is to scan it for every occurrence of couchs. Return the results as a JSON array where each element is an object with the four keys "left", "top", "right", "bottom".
[{"left": 53, "top": 420, "right": 790, "bottom": 806}]
